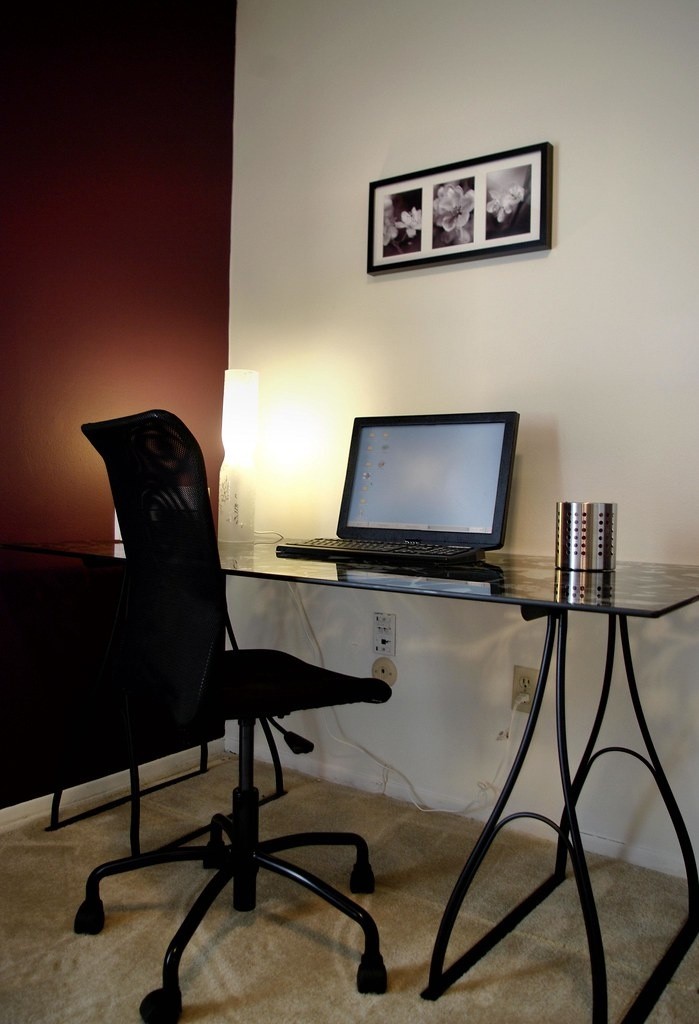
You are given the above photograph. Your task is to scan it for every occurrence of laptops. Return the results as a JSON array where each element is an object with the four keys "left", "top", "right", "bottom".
[{"left": 276, "top": 411, "right": 520, "bottom": 561}]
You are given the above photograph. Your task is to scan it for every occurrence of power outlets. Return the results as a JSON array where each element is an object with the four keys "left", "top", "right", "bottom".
[{"left": 510, "top": 664, "right": 539, "bottom": 713}]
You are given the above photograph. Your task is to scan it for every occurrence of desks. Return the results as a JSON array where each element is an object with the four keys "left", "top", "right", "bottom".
[{"left": 6, "top": 535, "right": 699, "bottom": 1024}]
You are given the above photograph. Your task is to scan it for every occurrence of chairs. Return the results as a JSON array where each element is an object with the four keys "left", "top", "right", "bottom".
[{"left": 72, "top": 409, "right": 387, "bottom": 1024}]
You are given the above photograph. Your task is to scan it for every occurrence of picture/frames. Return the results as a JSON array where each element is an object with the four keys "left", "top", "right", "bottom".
[{"left": 366, "top": 141, "right": 555, "bottom": 275}]
